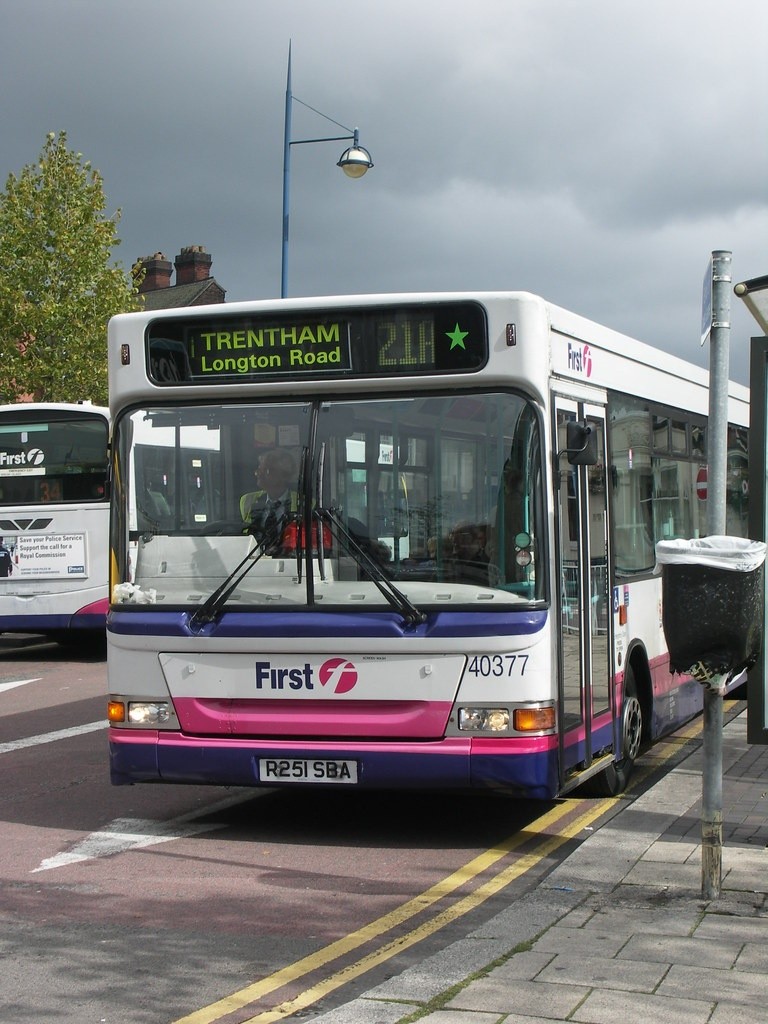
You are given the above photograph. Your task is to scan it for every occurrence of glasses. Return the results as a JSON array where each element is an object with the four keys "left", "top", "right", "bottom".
[{"left": 250, "top": 466, "right": 280, "bottom": 478}]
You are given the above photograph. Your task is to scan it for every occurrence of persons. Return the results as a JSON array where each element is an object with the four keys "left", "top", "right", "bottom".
[
  {"left": 427, "top": 536, "right": 448, "bottom": 558},
  {"left": 428, "top": 521, "right": 490, "bottom": 588},
  {"left": 240, "top": 450, "right": 317, "bottom": 533}
]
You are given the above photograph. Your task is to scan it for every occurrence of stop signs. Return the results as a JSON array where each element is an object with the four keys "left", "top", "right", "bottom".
[{"left": 696, "top": 468, "right": 708, "bottom": 501}]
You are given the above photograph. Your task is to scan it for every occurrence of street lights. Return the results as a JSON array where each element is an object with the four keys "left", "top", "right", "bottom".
[{"left": 281, "top": 129, "right": 375, "bottom": 299}]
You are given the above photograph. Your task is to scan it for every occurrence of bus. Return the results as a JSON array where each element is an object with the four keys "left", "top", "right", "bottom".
[
  {"left": 0, "top": 400, "right": 409, "bottom": 659},
  {"left": 106, "top": 292, "right": 748, "bottom": 799}
]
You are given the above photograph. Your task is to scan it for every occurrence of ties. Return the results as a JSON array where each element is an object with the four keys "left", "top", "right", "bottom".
[{"left": 262, "top": 501, "right": 281, "bottom": 549}]
